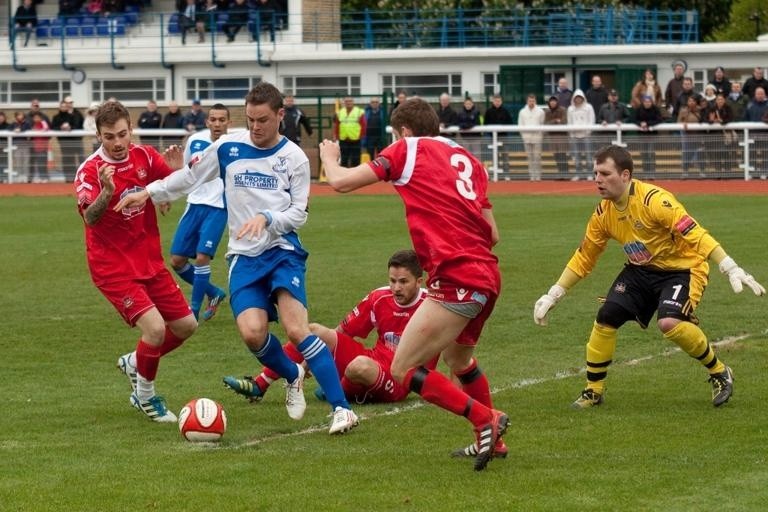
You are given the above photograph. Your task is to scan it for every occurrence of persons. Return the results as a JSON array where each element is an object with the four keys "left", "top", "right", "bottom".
[
  {"left": 137, "top": 100, "right": 161, "bottom": 154},
  {"left": 565, "top": 88, "right": 599, "bottom": 182},
  {"left": 222, "top": 249, "right": 440, "bottom": 406},
  {"left": 543, "top": 94, "right": 572, "bottom": 179},
  {"left": 112, "top": 82, "right": 361, "bottom": 437},
  {"left": 25, "top": 112, "right": 50, "bottom": 185},
  {"left": 433, "top": 92, "right": 456, "bottom": 133},
  {"left": 457, "top": 97, "right": 481, "bottom": 163},
  {"left": 64, "top": 95, "right": 86, "bottom": 165},
  {"left": 598, "top": 88, "right": 630, "bottom": 149},
  {"left": 552, "top": 77, "right": 572, "bottom": 109},
  {"left": 360, "top": 95, "right": 383, "bottom": 157},
  {"left": 707, "top": 66, "right": 731, "bottom": 99},
  {"left": 702, "top": 94, "right": 733, "bottom": 178},
  {"left": 703, "top": 84, "right": 718, "bottom": 101},
  {"left": 676, "top": 95, "right": 707, "bottom": 179},
  {"left": 161, "top": 103, "right": 184, "bottom": 151},
  {"left": 665, "top": 59, "right": 687, "bottom": 112},
  {"left": 740, "top": 67, "right": 768, "bottom": 100},
  {"left": 630, "top": 67, "right": 662, "bottom": 111},
  {"left": 50, "top": 101, "right": 78, "bottom": 183},
  {"left": 584, "top": 74, "right": 608, "bottom": 124},
  {"left": 517, "top": 92, "right": 546, "bottom": 181},
  {"left": 740, "top": 87, "right": 768, "bottom": 123},
  {"left": 333, "top": 96, "right": 365, "bottom": 167},
  {"left": 533, "top": 144, "right": 764, "bottom": 411},
  {"left": 20, "top": 99, "right": 52, "bottom": 183},
  {"left": 278, "top": 90, "right": 315, "bottom": 147},
  {"left": 82, "top": 106, "right": 98, "bottom": 160},
  {"left": 168, "top": 102, "right": 224, "bottom": 323},
  {"left": 672, "top": 76, "right": 702, "bottom": 122},
  {"left": 7, "top": 111, "right": 31, "bottom": 182},
  {"left": 0, "top": 111, "right": 11, "bottom": 184},
  {"left": 181, "top": 98, "right": 209, "bottom": 132},
  {"left": 70, "top": 99, "right": 205, "bottom": 432},
  {"left": 724, "top": 82, "right": 743, "bottom": 117},
  {"left": 0, "top": 1, "right": 287, "bottom": 49},
  {"left": 387, "top": 91, "right": 407, "bottom": 128},
  {"left": 481, "top": 94, "right": 512, "bottom": 182},
  {"left": 630, "top": 96, "right": 664, "bottom": 179},
  {"left": 318, "top": 97, "right": 511, "bottom": 472}
]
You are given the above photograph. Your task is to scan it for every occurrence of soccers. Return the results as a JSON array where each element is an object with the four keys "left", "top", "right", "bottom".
[{"left": 178, "top": 399, "right": 227, "bottom": 441}]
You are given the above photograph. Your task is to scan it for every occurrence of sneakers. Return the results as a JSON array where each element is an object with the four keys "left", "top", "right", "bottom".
[
  {"left": 451, "top": 437, "right": 507, "bottom": 459},
  {"left": 116, "top": 354, "right": 139, "bottom": 391},
  {"left": 203, "top": 287, "right": 226, "bottom": 321},
  {"left": 474, "top": 410, "right": 508, "bottom": 468},
  {"left": 328, "top": 406, "right": 358, "bottom": 434},
  {"left": 707, "top": 366, "right": 733, "bottom": 405},
  {"left": 129, "top": 389, "right": 177, "bottom": 423},
  {"left": 572, "top": 388, "right": 602, "bottom": 410},
  {"left": 222, "top": 374, "right": 263, "bottom": 404},
  {"left": 285, "top": 363, "right": 306, "bottom": 421}
]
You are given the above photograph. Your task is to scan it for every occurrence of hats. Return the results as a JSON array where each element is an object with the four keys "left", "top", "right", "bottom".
[{"left": 607, "top": 89, "right": 618, "bottom": 95}]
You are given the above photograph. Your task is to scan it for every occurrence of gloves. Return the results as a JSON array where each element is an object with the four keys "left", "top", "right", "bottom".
[
  {"left": 720, "top": 256, "right": 766, "bottom": 298},
  {"left": 532, "top": 284, "right": 565, "bottom": 326}
]
